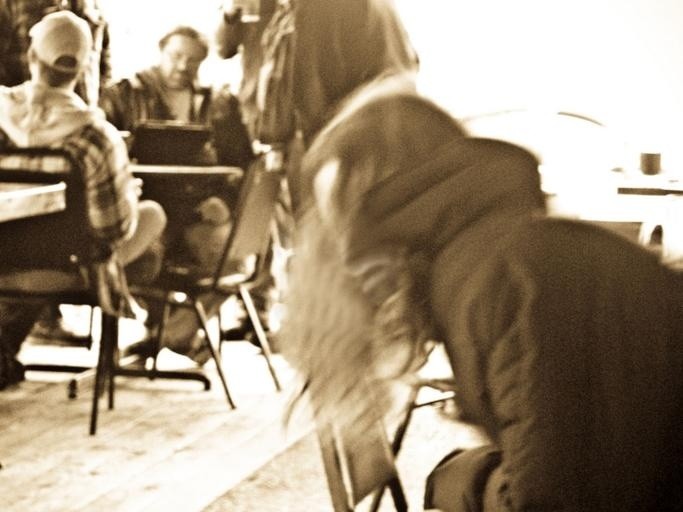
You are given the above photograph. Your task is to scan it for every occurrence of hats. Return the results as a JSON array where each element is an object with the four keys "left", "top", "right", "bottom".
[{"left": 29, "top": 10, "right": 93, "bottom": 74}]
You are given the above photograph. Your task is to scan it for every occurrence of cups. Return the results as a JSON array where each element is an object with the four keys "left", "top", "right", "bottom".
[{"left": 639, "top": 151, "right": 662, "bottom": 176}]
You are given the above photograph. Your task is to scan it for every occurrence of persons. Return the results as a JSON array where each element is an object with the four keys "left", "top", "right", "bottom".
[{"left": 308, "top": 93, "right": 682, "bottom": 511}]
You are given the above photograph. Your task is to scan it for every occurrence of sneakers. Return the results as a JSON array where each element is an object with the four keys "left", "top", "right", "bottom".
[{"left": 28, "top": 321, "right": 88, "bottom": 345}]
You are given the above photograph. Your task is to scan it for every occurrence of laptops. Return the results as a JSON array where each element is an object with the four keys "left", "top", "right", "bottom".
[{"left": 125, "top": 121, "right": 214, "bottom": 165}]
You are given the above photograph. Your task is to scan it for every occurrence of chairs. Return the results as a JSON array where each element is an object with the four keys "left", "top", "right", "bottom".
[{"left": 0, "top": 152, "right": 282, "bottom": 436}]
